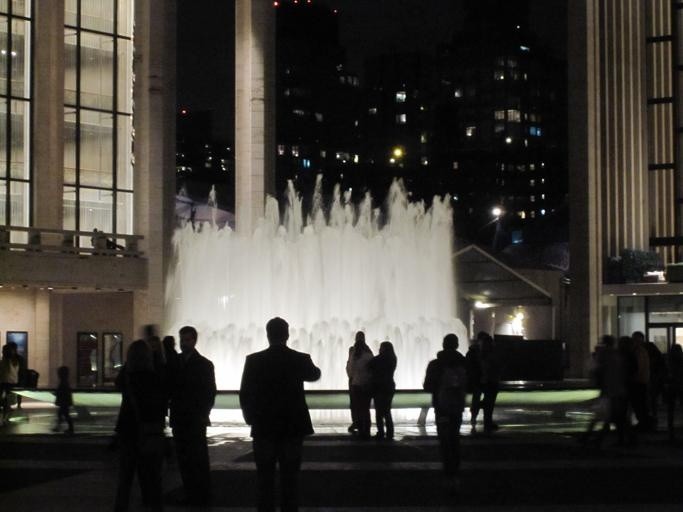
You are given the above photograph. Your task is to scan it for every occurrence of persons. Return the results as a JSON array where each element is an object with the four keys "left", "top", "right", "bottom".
[
  {"left": 239, "top": 316, "right": 322, "bottom": 511},
  {"left": 344, "top": 336, "right": 375, "bottom": 440},
  {"left": 97, "top": 231, "right": 112, "bottom": 256},
  {"left": 0, "top": 341, "right": 26, "bottom": 408},
  {"left": 90, "top": 227, "right": 97, "bottom": 246},
  {"left": 48, "top": 366, "right": 74, "bottom": 434},
  {"left": 584, "top": 332, "right": 683, "bottom": 435},
  {"left": 347, "top": 330, "right": 372, "bottom": 432},
  {"left": 113, "top": 323, "right": 218, "bottom": 512},
  {"left": 466, "top": 330, "right": 505, "bottom": 431},
  {"left": 422, "top": 333, "right": 474, "bottom": 480},
  {"left": 364, "top": 340, "right": 397, "bottom": 439}
]
